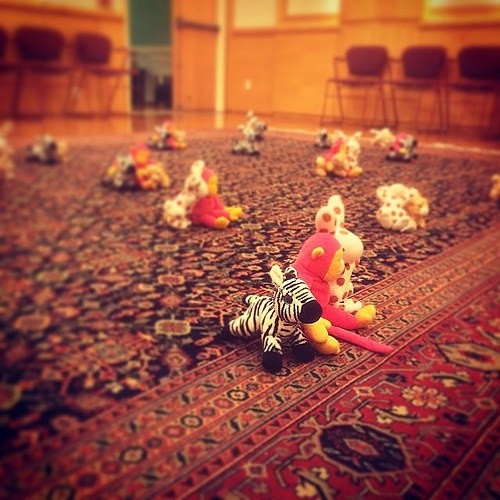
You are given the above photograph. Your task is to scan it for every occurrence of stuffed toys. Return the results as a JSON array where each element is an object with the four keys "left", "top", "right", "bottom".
[
  {"left": 233, "top": 111, "right": 266, "bottom": 155},
  {"left": 314, "top": 127, "right": 363, "bottom": 177},
  {"left": 223, "top": 195, "right": 393, "bottom": 374},
  {"left": 102, "top": 122, "right": 187, "bottom": 189},
  {"left": 371, "top": 128, "right": 419, "bottom": 163},
  {"left": 376, "top": 183, "right": 430, "bottom": 232},
  {"left": 161, "top": 160, "right": 242, "bottom": 230},
  {"left": 24, "top": 136, "right": 64, "bottom": 163}
]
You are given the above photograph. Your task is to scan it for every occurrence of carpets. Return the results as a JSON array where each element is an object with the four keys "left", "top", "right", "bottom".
[{"left": 0, "top": 126, "right": 500, "bottom": 500}]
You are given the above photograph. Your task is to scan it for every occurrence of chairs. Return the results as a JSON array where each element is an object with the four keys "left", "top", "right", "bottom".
[
  {"left": 447, "top": 44, "right": 500, "bottom": 138},
  {"left": 320, "top": 47, "right": 400, "bottom": 125},
  {"left": 0, "top": 26, "right": 72, "bottom": 119},
  {"left": 383, "top": 47, "right": 448, "bottom": 132},
  {"left": 69, "top": 31, "right": 141, "bottom": 120}
]
